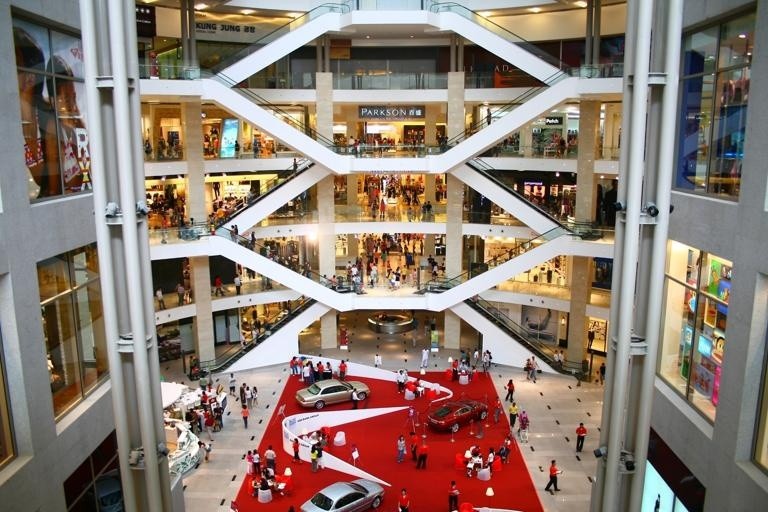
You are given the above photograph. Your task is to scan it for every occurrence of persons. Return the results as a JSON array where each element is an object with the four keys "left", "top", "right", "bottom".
[
  {"left": 369, "top": 310, "right": 604, "bottom": 444},
  {"left": 486, "top": 108, "right": 491, "bottom": 125},
  {"left": 603, "top": 179, "right": 618, "bottom": 226},
  {"left": 150, "top": 257, "right": 348, "bottom": 463},
  {"left": 652, "top": 493, "right": 660, "bottom": 512},
  {"left": 8, "top": 30, "right": 60, "bottom": 198},
  {"left": 143, "top": 134, "right": 379, "bottom": 294},
  {"left": 373, "top": 136, "right": 578, "bottom": 307},
  {"left": 245, "top": 423, "right": 589, "bottom": 510},
  {"left": 40, "top": 54, "right": 90, "bottom": 201}
]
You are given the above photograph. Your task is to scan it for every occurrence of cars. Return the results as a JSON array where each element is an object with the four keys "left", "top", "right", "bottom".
[
  {"left": 299, "top": 478, "right": 386, "bottom": 512},
  {"left": 426, "top": 399, "right": 490, "bottom": 433},
  {"left": 296, "top": 378, "right": 371, "bottom": 410}
]
